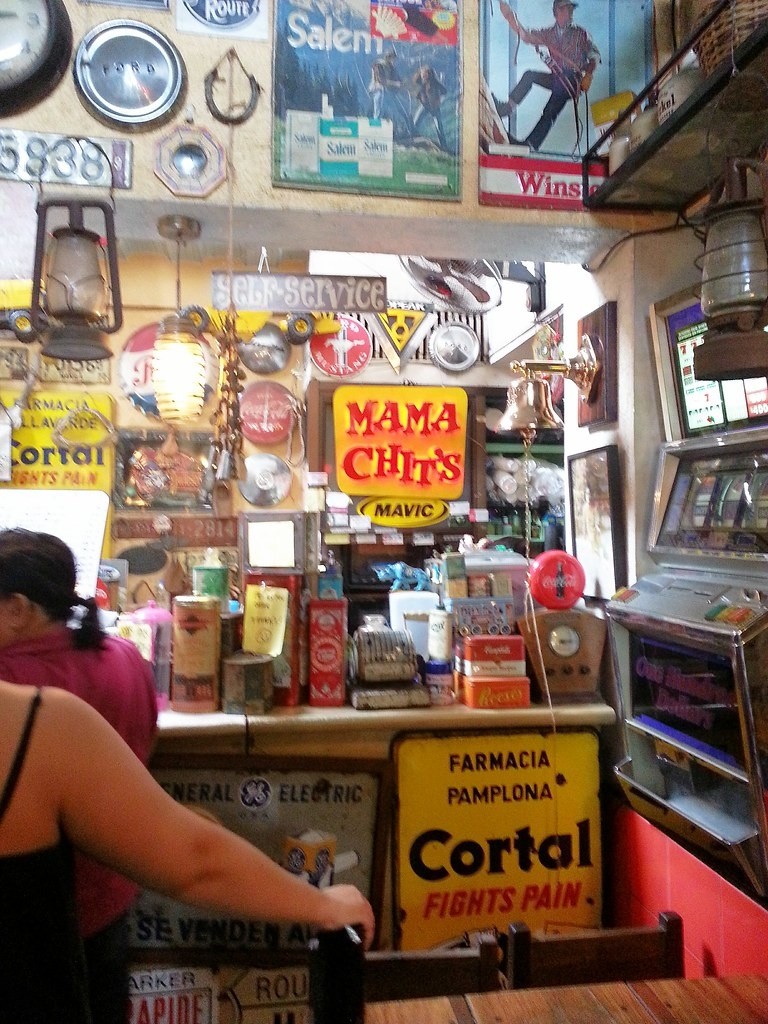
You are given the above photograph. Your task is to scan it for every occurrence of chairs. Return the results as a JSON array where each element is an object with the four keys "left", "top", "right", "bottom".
[
  {"left": 507, "top": 910, "right": 685, "bottom": 990},
  {"left": 307, "top": 917, "right": 501, "bottom": 1024}
]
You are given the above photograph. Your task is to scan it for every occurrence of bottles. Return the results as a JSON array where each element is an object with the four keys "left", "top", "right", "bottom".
[{"left": 488, "top": 507, "right": 543, "bottom": 540}]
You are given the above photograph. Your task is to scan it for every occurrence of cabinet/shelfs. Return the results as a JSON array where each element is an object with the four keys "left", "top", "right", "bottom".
[{"left": 581, "top": 1, "right": 768, "bottom": 219}]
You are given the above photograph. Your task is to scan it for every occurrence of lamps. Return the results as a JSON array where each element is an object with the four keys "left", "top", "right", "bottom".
[
  {"left": 149, "top": 212, "right": 214, "bottom": 422},
  {"left": 24, "top": 132, "right": 125, "bottom": 363},
  {"left": 688, "top": 71, "right": 768, "bottom": 382}
]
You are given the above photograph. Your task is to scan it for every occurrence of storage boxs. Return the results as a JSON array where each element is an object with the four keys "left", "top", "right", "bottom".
[
  {"left": 448, "top": 669, "right": 531, "bottom": 711},
  {"left": 449, "top": 632, "right": 527, "bottom": 678}
]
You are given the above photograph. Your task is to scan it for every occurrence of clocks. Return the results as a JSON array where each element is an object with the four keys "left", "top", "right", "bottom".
[{"left": 0, "top": 0, "right": 74, "bottom": 120}]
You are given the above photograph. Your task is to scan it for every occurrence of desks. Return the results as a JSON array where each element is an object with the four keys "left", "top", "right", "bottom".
[{"left": 363, "top": 974, "right": 768, "bottom": 1024}]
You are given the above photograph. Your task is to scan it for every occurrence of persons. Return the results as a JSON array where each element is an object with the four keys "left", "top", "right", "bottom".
[
  {"left": 0, "top": 527, "right": 159, "bottom": 1024},
  {"left": 413, "top": 65, "right": 448, "bottom": 149},
  {"left": 368, "top": 50, "right": 402, "bottom": 119},
  {"left": 490, "top": 0, "right": 602, "bottom": 153},
  {"left": 0, "top": 678, "right": 377, "bottom": 1024}
]
